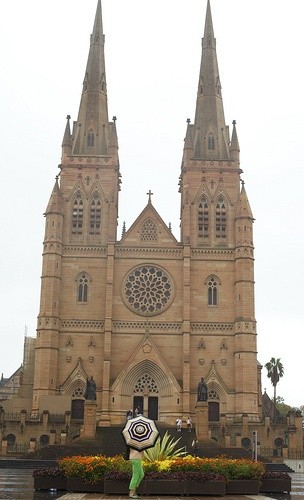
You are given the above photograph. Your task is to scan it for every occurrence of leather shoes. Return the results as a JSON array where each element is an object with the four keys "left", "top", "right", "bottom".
[{"left": 129, "top": 494, "right": 142, "bottom": 499}]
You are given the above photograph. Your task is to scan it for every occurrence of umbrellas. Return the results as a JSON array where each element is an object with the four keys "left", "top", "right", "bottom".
[{"left": 121, "top": 415, "right": 160, "bottom": 452}]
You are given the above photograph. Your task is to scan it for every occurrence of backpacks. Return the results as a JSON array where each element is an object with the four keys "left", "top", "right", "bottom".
[{"left": 123, "top": 446, "right": 130, "bottom": 460}]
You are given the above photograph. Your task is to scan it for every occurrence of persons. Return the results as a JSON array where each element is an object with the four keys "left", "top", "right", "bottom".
[
  {"left": 129, "top": 445, "right": 145, "bottom": 499},
  {"left": 175, "top": 417, "right": 182, "bottom": 433},
  {"left": 197, "top": 377, "right": 207, "bottom": 402},
  {"left": 186, "top": 416, "right": 192, "bottom": 434},
  {"left": 87, "top": 375, "right": 97, "bottom": 400}
]
